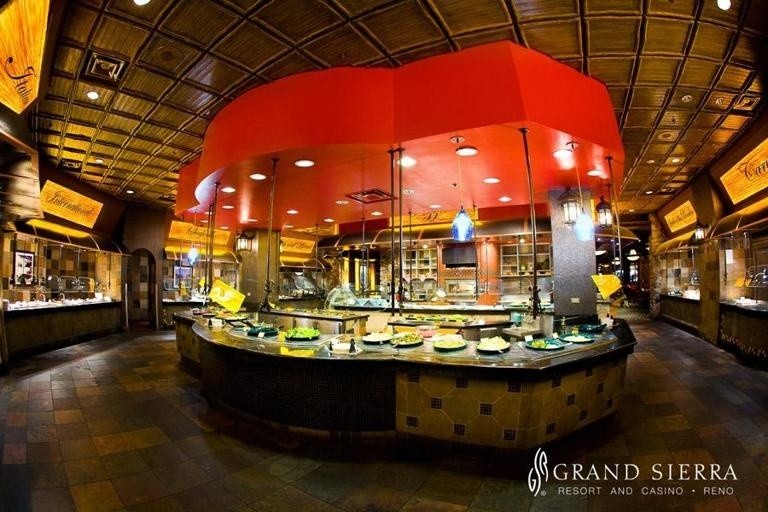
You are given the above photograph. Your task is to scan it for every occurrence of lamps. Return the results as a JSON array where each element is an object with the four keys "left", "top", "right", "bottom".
[
  {"left": 693, "top": 219, "right": 708, "bottom": 240},
  {"left": 450, "top": 134, "right": 474, "bottom": 242},
  {"left": 565, "top": 142, "right": 613, "bottom": 242}
]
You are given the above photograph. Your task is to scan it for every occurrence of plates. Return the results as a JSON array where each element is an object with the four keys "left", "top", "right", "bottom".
[
  {"left": 362, "top": 333, "right": 392, "bottom": 343},
  {"left": 477, "top": 340, "right": 511, "bottom": 352},
  {"left": 559, "top": 332, "right": 596, "bottom": 343},
  {"left": 390, "top": 331, "right": 424, "bottom": 346},
  {"left": 433, "top": 338, "right": 467, "bottom": 349},
  {"left": 526, "top": 337, "right": 565, "bottom": 350}
]
